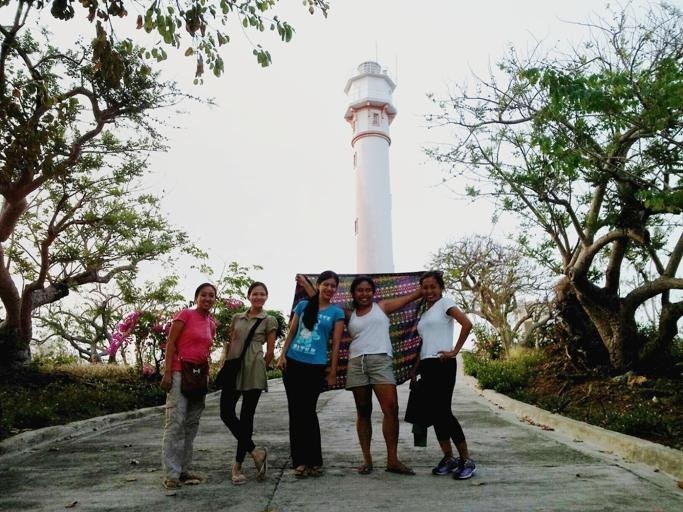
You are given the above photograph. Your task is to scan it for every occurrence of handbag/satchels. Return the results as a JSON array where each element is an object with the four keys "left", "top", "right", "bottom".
[
  {"left": 180, "top": 361, "right": 208, "bottom": 397},
  {"left": 214, "top": 358, "right": 242, "bottom": 391}
]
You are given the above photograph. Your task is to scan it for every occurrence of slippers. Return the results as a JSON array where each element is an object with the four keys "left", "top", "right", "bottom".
[
  {"left": 163, "top": 480, "right": 181, "bottom": 490},
  {"left": 255, "top": 447, "right": 268, "bottom": 482},
  {"left": 231, "top": 474, "right": 247, "bottom": 485},
  {"left": 181, "top": 476, "right": 203, "bottom": 482}
]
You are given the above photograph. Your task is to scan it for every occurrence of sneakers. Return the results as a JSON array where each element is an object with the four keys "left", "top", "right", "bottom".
[
  {"left": 296, "top": 465, "right": 326, "bottom": 477},
  {"left": 454, "top": 457, "right": 476, "bottom": 479},
  {"left": 433, "top": 455, "right": 459, "bottom": 475}
]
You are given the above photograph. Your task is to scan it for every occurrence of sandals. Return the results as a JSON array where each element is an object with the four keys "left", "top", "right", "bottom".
[
  {"left": 358, "top": 460, "right": 373, "bottom": 474},
  {"left": 385, "top": 462, "right": 416, "bottom": 475}
]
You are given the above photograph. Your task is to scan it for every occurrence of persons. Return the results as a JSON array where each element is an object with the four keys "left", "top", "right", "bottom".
[
  {"left": 409, "top": 272, "right": 476, "bottom": 481},
  {"left": 158, "top": 282, "right": 217, "bottom": 490},
  {"left": 277, "top": 270, "right": 346, "bottom": 477},
  {"left": 295, "top": 272, "right": 426, "bottom": 477},
  {"left": 219, "top": 281, "right": 279, "bottom": 483}
]
